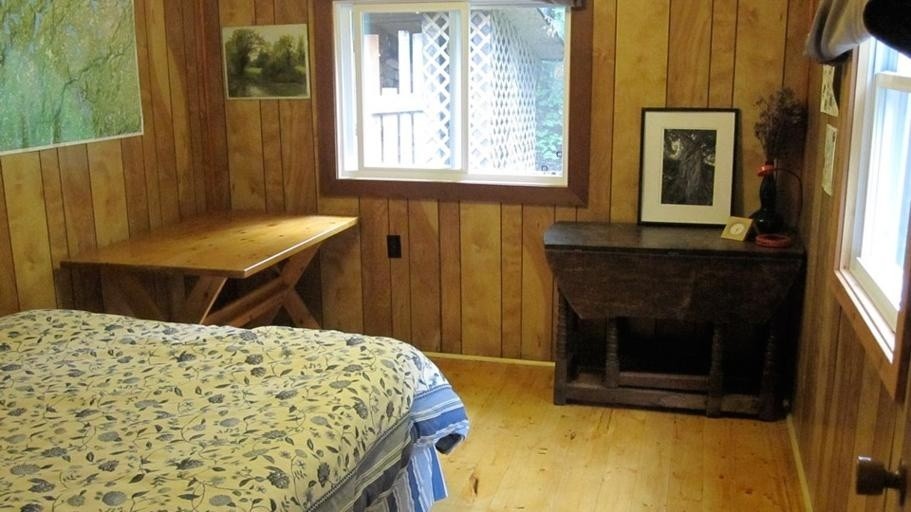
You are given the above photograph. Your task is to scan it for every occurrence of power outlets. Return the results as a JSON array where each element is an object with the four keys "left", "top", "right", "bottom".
[{"left": 387, "top": 235, "right": 401, "bottom": 259}]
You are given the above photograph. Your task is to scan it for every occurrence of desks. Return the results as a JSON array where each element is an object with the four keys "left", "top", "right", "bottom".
[{"left": 61, "top": 211, "right": 358, "bottom": 328}]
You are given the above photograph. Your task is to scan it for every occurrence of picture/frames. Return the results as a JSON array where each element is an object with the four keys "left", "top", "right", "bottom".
[
  {"left": 638, "top": 108, "right": 739, "bottom": 228},
  {"left": 222, "top": 23, "right": 313, "bottom": 100}
]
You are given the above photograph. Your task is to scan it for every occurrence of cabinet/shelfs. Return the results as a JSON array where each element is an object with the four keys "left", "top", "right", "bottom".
[{"left": 542, "top": 221, "right": 806, "bottom": 424}]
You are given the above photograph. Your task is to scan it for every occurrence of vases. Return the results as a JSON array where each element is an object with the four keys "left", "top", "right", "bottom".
[{"left": 752, "top": 155, "right": 782, "bottom": 235}]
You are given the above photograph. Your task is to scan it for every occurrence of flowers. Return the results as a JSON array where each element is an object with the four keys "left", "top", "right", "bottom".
[{"left": 754, "top": 87, "right": 806, "bottom": 158}]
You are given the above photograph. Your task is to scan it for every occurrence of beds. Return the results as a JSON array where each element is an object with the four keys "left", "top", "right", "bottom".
[{"left": 1, "top": 310, "right": 470, "bottom": 511}]
